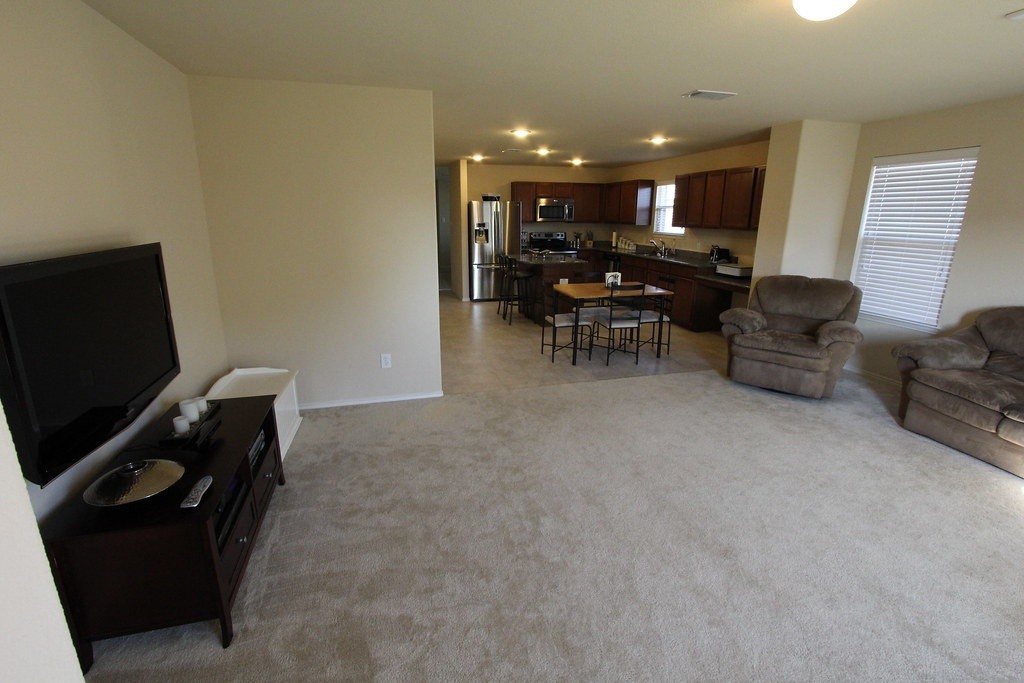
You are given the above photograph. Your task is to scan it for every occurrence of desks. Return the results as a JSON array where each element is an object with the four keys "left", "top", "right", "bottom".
[{"left": 553, "top": 280, "right": 674, "bottom": 366}]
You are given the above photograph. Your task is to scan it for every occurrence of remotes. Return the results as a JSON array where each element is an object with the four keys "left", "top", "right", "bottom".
[{"left": 181, "top": 476, "right": 213, "bottom": 509}]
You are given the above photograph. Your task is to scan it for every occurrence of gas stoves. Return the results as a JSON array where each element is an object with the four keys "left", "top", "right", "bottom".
[{"left": 530, "top": 232, "right": 577, "bottom": 250}]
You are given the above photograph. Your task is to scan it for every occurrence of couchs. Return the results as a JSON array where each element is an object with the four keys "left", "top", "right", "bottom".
[
  {"left": 891, "top": 306, "right": 1024, "bottom": 478},
  {"left": 720, "top": 275, "right": 862, "bottom": 400}
]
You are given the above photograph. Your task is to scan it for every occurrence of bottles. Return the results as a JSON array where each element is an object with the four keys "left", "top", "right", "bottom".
[
  {"left": 570, "top": 240, "right": 576, "bottom": 246},
  {"left": 521, "top": 227, "right": 528, "bottom": 247},
  {"left": 618, "top": 234, "right": 636, "bottom": 250}
]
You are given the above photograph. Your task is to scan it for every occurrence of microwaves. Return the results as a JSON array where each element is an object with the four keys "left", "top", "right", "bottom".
[{"left": 536, "top": 197, "right": 575, "bottom": 222}]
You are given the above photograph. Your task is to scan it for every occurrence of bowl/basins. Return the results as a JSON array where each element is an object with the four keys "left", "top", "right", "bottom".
[
  {"left": 482, "top": 195, "right": 499, "bottom": 202},
  {"left": 560, "top": 278, "right": 570, "bottom": 286}
]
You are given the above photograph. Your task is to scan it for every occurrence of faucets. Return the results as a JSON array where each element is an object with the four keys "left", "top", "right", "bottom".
[{"left": 649, "top": 239, "right": 665, "bottom": 255}]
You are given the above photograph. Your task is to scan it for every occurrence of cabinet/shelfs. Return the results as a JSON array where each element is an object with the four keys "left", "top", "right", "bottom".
[
  {"left": 672, "top": 165, "right": 766, "bottom": 232},
  {"left": 511, "top": 179, "right": 655, "bottom": 226},
  {"left": 577, "top": 251, "right": 732, "bottom": 333},
  {"left": 40, "top": 394, "right": 285, "bottom": 676}
]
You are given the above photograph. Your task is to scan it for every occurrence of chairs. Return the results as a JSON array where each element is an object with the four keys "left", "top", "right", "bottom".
[{"left": 541, "top": 272, "right": 675, "bottom": 366}]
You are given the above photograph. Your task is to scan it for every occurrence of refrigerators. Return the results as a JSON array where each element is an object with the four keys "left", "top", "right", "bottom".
[{"left": 468, "top": 200, "right": 520, "bottom": 299}]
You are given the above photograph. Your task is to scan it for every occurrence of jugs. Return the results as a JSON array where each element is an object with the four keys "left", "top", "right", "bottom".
[{"left": 707, "top": 244, "right": 721, "bottom": 261}]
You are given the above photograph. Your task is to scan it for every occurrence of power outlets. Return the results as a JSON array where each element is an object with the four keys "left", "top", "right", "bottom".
[{"left": 381, "top": 354, "right": 391, "bottom": 369}]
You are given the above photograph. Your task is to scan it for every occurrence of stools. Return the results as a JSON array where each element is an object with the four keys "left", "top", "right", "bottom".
[{"left": 497, "top": 254, "right": 536, "bottom": 326}]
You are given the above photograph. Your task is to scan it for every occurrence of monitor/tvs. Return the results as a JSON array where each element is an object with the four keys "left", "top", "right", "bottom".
[{"left": 0, "top": 242, "right": 184, "bottom": 492}]
